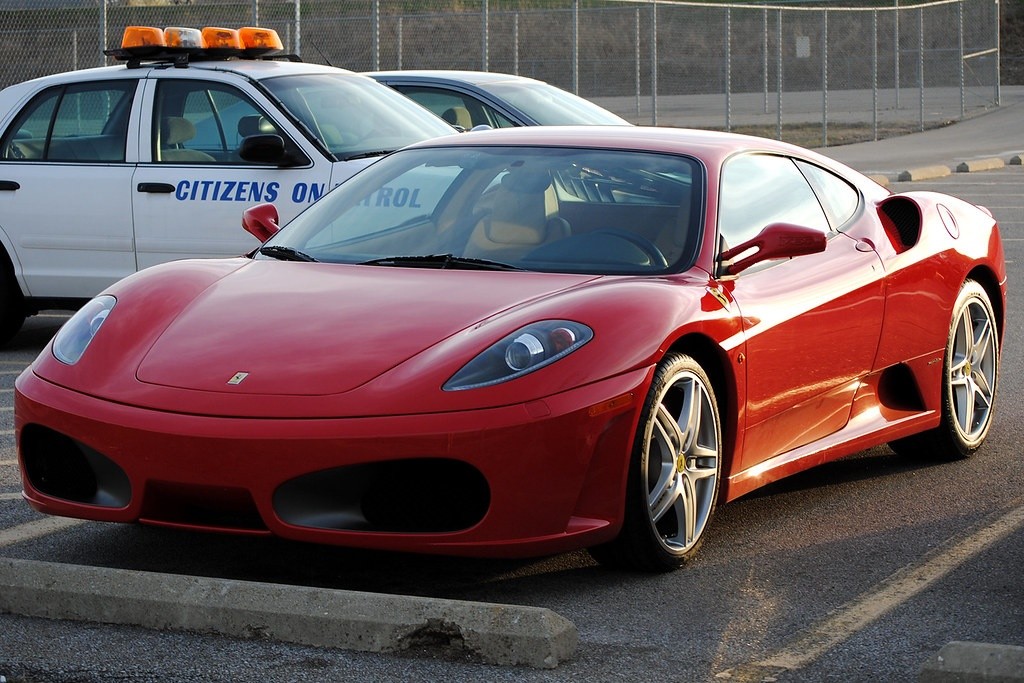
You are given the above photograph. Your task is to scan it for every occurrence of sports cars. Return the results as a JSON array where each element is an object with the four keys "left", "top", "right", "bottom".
[{"left": 14, "top": 123, "right": 1007, "bottom": 578}]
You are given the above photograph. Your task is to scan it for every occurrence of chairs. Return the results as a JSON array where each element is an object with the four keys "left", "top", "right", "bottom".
[
  {"left": 234, "top": 115, "right": 276, "bottom": 162},
  {"left": 462, "top": 177, "right": 572, "bottom": 259},
  {"left": 161, "top": 116, "right": 216, "bottom": 161},
  {"left": 443, "top": 107, "right": 473, "bottom": 129}
]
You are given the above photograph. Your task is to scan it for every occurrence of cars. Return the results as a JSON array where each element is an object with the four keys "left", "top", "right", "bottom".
[
  {"left": 0, "top": 24, "right": 507, "bottom": 356},
  {"left": 177, "top": 69, "right": 694, "bottom": 207}
]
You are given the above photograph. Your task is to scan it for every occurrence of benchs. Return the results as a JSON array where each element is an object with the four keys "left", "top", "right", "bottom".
[{"left": 15, "top": 132, "right": 121, "bottom": 159}]
